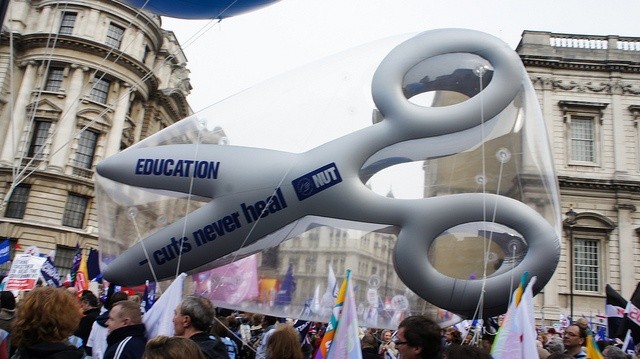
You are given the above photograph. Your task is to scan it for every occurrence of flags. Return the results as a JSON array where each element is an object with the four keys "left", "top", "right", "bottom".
[
  {"left": 142, "top": 272, "right": 188, "bottom": 340},
  {"left": 71, "top": 240, "right": 81, "bottom": 287},
  {"left": 365, "top": 290, "right": 384, "bottom": 326},
  {"left": 621, "top": 329, "right": 635, "bottom": 357},
  {"left": 517, "top": 275, "right": 540, "bottom": 359},
  {"left": 605, "top": 280, "right": 628, "bottom": 340},
  {"left": 322, "top": 264, "right": 340, "bottom": 300},
  {"left": 596, "top": 315, "right": 607, "bottom": 341},
  {"left": 63, "top": 273, "right": 71, "bottom": 286},
  {"left": 585, "top": 329, "right": 603, "bottom": 359},
  {"left": 74, "top": 250, "right": 89, "bottom": 297},
  {"left": 392, "top": 330, "right": 399, "bottom": 342},
  {"left": 144, "top": 279, "right": 155, "bottom": 312},
  {"left": 616, "top": 281, "right": 640, "bottom": 344},
  {"left": 313, "top": 269, "right": 363, "bottom": 359},
  {"left": 292, "top": 299, "right": 313, "bottom": 345},
  {"left": 0, "top": 239, "right": 10, "bottom": 266},
  {"left": 273, "top": 262, "right": 296, "bottom": 308},
  {"left": 364, "top": 286, "right": 410, "bottom": 327},
  {"left": 191, "top": 255, "right": 259, "bottom": 306},
  {"left": 89, "top": 274, "right": 108, "bottom": 303},
  {"left": 36, "top": 252, "right": 59, "bottom": 286},
  {"left": 489, "top": 270, "right": 528, "bottom": 359}
]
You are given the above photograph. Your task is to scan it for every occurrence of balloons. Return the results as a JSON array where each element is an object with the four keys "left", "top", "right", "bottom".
[{"left": 118, "top": 0, "right": 283, "bottom": 19}]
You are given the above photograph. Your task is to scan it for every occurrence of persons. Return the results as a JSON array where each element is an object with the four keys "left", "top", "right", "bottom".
[
  {"left": 250, "top": 314, "right": 265, "bottom": 337},
  {"left": 366, "top": 327, "right": 381, "bottom": 344},
  {"left": 563, "top": 325, "right": 586, "bottom": 359},
  {"left": 575, "top": 318, "right": 590, "bottom": 330},
  {"left": 10, "top": 286, "right": 89, "bottom": 358},
  {"left": 555, "top": 333, "right": 561, "bottom": 338},
  {"left": 378, "top": 330, "right": 400, "bottom": 359},
  {"left": 0, "top": 329, "right": 9, "bottom": 359},
  {"left": 86, "top": 292, "right": 128, "bottom": 359},
  {"left": 545, "top": 336, "right": 565, "bottom": 353},
  {"left": 208, "top": 318, "right": 239, "bottom": 359},
  {"left": 362, "top": 337, "right": 378, "bottom": 359},
  {"left": 602, "top": 346, "right": 628, "bottom": 358},
  {"left": 632, "top": 343, "right": 640, "bottom": 359},
  {"left": 395, "top": 315, "right": 441, "bottom": 359},
  {"left": 546, "top": 333, "right": 552, "bottom": 339},
  {"left": 595, "top": 339, "right": 609, "bottom": 352},
  {"left": 225, "top": 316, "right": 242, "bottom": 352},
  {"left": 538, "top": 334, "right": 547, "bottom": 348},
  {"left": 442, "top": 343, "right": 479, "bottom": 359},
  {"left": 545, "top": 352, "right": 576, "bottom": 359},
  {"left": 260, "top": 314, "right": 276, "bottom": 337},
  {"left": 244, "top": 311, "right": 254, "bottom": 326},
  {"left": 104, "top": 300, "right": 146, "bottom": 359},
  {"left": 142, "top": 336, "right": 204, "bottom": 359},
  {"left": 441, "top": 324, "right": 489, "bottom": 346},
  {"left": 75, "top": 292, "right": 99, "bottom": 356},
  {"left": 172, "top": 294, "right": 231, "bottom": 359},
  {"left": 255, "top": 322, "right": 305, "bottom": 359},
  {"left": 0, "top": 290, "right": 16, "bottom": 332},
  {"left": 468, "top": 346, "right": 494, "bottom": 359},
  {"left": 548, "top": 328, "right": 556, "bottom": 334}
]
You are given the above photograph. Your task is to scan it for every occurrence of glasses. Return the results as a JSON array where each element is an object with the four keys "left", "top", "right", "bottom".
[
  {"left": 395, "top": 340, "right": 408, "bottom": 347},
  {"left": 563, "top": 331, "right": 581, "bottom": 337}
]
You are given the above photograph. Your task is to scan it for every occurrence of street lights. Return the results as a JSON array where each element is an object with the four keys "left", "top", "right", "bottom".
[{"left": 565, "top": 204, "right": 577, "bottom": 326}]
